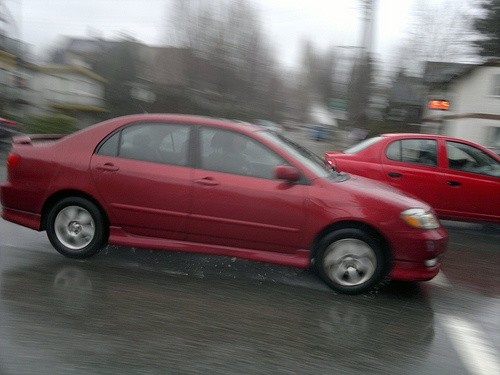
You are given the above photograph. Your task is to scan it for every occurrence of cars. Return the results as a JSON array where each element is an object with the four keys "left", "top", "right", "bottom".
[
  {"left": 1, "top": 113, "right": 448, "bottom": 296},
  {"left": 326, "top": 134, "right": 499, "bottom": 226}
]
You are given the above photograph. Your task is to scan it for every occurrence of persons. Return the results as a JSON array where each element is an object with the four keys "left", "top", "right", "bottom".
[
  {"left": 429, "top": 142, "right": 472, "bottom": 172},
  {"left": 218, "top": 134, "right": 261, "bottom": 176}
]
